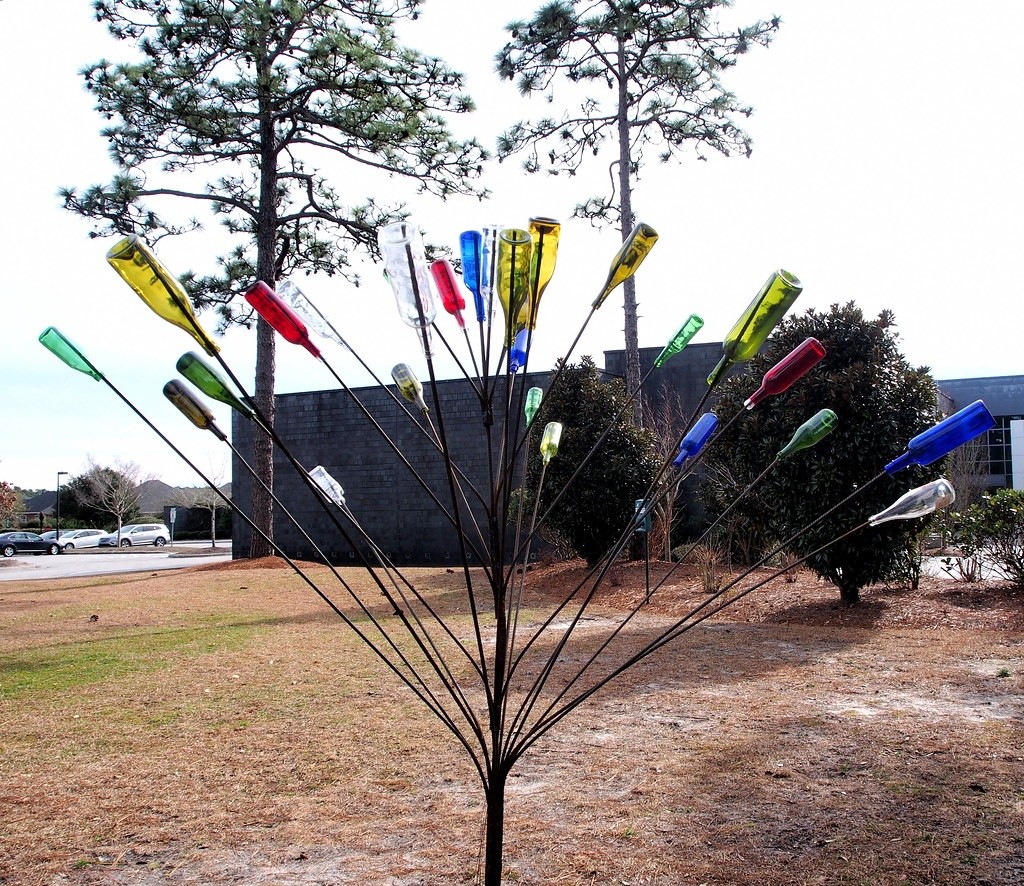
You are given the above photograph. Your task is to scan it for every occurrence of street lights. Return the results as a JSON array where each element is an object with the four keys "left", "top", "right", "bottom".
[{"left": 56, "top": 472, "right": 68, "bottom": 536}]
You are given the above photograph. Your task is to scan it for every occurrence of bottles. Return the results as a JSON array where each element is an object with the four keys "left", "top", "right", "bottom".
[
  {"left": 743, "top": 337, "right": 827, "bottom": 411},
  {"left": 776, "top": 408, "right": 839, "bottom": 461},
  {"left": 634, "top": 498, "right": 652, "bottom": 532},
  {"left": 525, "top": 387, "right": 543, "bottom": 426},
  {"left": 307, "top": 465, "right": 346, "bottom": 506},
  {"left": 391, "top": 363, "right": 430, "bottom": 415},
  {"left": 163, "top": 378, "right": 227, "bottom": 441},
  {"left": 105, "top": 233, "right": 221, "bottom": 357},
  {"left": 673, "top": 412, "right": 719, "bottom": 468},
  {"left": 591, "top": 221, "right": 660, "bottom": 310},
  {"left": 540, "top": 422, "right": 563, "bottom": 466},
  {"left": 867, "top": 478, "right": 956, "bottom": 526},
  {"left": 276, "top": 280, "right": 348, "bottom": 349},
  {"left": 378, "top": 215, "right": 562, "bottom": 375},
  {"left": 885, "top": 399, "right": 998, "bottom": 475},
  {"left": 706, "top": 268, "right": 803, "bottom": 386},
  {"left": 176, "top": 351, "right": 257, "bottom": 421},
  {"left": 244, "top": 280, "right": 320, "bottom": 358},
  {"left": 653, "top": 314, "right": 704, "bottom": 368},
  {"left": 38, "top": 327, "right": 104, "bottom": 382}
]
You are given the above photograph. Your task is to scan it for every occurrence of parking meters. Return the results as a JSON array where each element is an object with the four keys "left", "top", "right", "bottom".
[
  {"left": 633, "top": 499, "right": 651, "bottom": 601},
  {"left": 170, "top": 508, "right": 176, "bottom": 546}
]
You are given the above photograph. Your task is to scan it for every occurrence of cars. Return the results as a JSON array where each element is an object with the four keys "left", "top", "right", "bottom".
[
  {"left": 99, "top": 523, "right": 170, "bottom": 548},
  {"left": 40, "top": 531, "right": 69, "bottom": 541},
  {"left": 0, "top": 532, "right": 61, "bottom": 557},
  {"left": 59, "top": 529, "right": 109, "bottom": 549}
]
